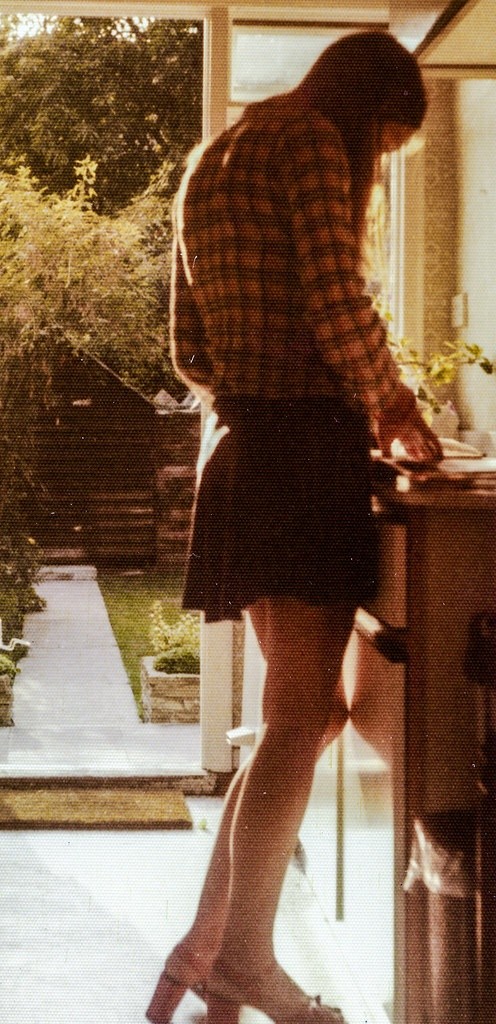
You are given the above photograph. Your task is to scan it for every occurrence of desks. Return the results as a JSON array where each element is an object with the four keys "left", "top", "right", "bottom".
[{"left": 335, "top": 441, "right": 494, "bottom": 1022}]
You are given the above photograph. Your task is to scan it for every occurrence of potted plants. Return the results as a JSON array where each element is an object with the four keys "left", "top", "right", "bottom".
[
  {"left": 140, "top": 602, "right": 204, "bottom": 724},
  {"left": 396, "top": 340, "right": 496, "bottom": 443}
]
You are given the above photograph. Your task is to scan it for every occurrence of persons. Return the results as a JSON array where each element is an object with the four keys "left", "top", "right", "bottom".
[{"left": 144, "top": 30, "right": 443, "bottom": 1024}]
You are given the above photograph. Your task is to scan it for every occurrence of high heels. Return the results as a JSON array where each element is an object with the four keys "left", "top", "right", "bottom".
[
  {"left": 145, "top": 943, "right": 214, "bottom": 1024},
  {"left": 206, "top": 955, "right": 348, "bottom": 1024}
]
